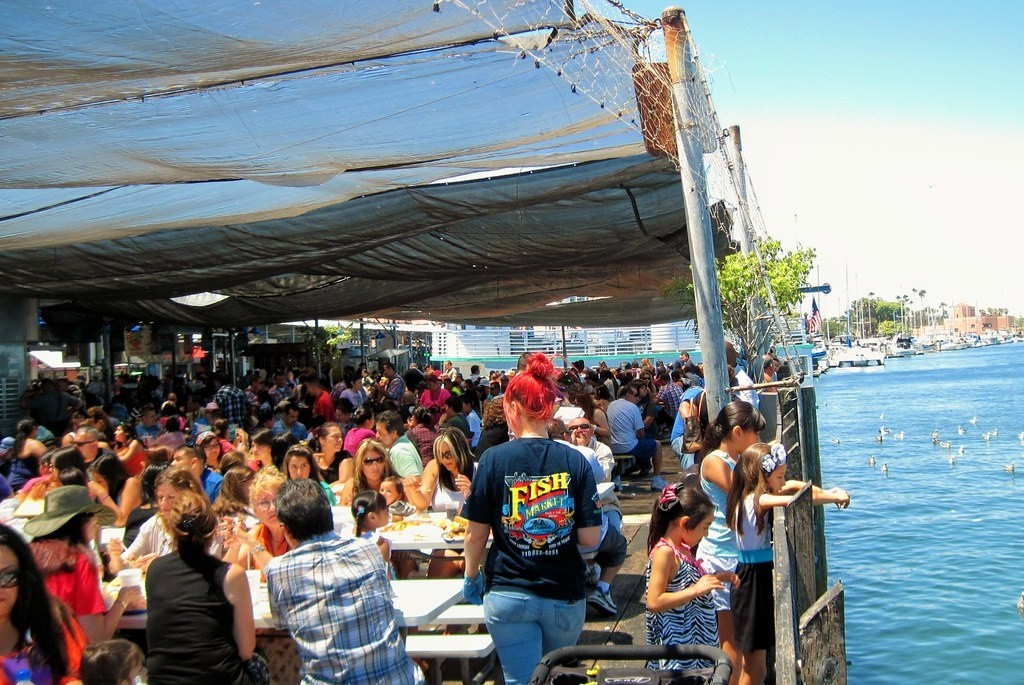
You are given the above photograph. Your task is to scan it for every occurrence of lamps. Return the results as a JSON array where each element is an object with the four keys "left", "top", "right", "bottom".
[
  {"left": 375, "top": 329, "right": 386, "bottom": 338},
  {"left": 249, "top": 326, "right": 261, "bottom": 335}
]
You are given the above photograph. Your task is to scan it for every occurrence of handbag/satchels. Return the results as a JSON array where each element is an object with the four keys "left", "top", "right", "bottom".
[{"left": 243, "top": 652, "right": 270, "bottom": 685}]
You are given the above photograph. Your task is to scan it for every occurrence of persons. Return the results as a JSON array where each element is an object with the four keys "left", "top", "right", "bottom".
[
  {"left": 670, "top": 341, "right": 780, "bottom": 478},
  {"left": 80, "top": 639, "right": 149, "bottom": 685},
  {"left": 402, "top": 426, "right": 488, "bottom": 635},
  {"left": 264, "top": 478, "right": 426, "bottom": 685},
  {"left": 645, "top": 483, "right": 741, "bottom": 685},
  {"left": 222, "top": 465, "right": 302, "bottom": 685},
  {"left": 145, "top": 491, "right": 270, "bottom": 685},
  {"left": 0, "top": 350, "right": 694, "bottom": 614},
  {"left": 23, "top": 485, "right": 142, "bottom": 644},
  {"left": 459, "top": 353, "right": 602, "bottom": 685},
  {"left": 725, "top": 444, "right": 850, "bottom": 685},
  {"left": 697, "top": 401, "right": 767, "bottom": 685},
  {"left": 338, "top": 438, "right": 428, "bottom": 672},
  {"left": 0, "top": 523, "right": 87, "bottom": 685}
]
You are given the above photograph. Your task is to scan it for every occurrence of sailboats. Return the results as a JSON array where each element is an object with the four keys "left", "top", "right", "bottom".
[{"left": 808, "top": 264, "right": 1023, "bottom": 377}]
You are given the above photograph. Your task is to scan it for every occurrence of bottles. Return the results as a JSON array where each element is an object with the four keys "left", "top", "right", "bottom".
[{"left": 16, "top": 670, "right": 36, "bottom": 685}]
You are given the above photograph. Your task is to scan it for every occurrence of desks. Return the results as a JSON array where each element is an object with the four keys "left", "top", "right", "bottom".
[
  {"left": 116, "top": 579, "right": 149, "bottom": 628},
  {"left": 253, "top": 577, "right": 467, "bottom": 628},
  {"left": 596, "top": 482, "right": 615, "bottom": 500},
  {"left": 377, "top": 512, "right": 494, "bottom": 550}
]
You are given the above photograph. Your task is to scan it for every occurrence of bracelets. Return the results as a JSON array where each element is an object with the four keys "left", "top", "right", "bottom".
[
  {"left": 114, "top": 599, "right": 128, "bottom": 608},
  {"left": 100, "top": 494, "right": 109, "bottom": 503},
  {"left": 251, "top": 544, "right": 265, "bottom": 554}
]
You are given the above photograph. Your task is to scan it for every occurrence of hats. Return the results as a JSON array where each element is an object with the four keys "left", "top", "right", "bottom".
[{"left": 23, "top": 485, "right": 118, "bottom": 538}]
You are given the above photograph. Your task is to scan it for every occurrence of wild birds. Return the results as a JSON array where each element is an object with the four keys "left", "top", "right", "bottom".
[
  {"left": 958, "top": 426, "right": 966, "bottom": 435},
  {"left": 949, "top": 455, "right": 954, "bottom": 464},
  {"left": 832, "top": 437, "right": 840, "bottom": 443},
  {"left": 1018, "top": 432, "right": 1024, "bottom": 440},
  {"left": 1017, "top": 591, "right": 1024, "bottom": 612},
  {"left": 983, "top": 432, "right": 991, "bottom": 440},
  {"left": 970, "top": 417, "right": 976, "bottom": 424},
  {"left": 880, "top": 413, "right": 885, "bottom": 419},
  {"left": 879, "top": 426, "right": 890, "bottom": 435},
  {"left": 1003, "top": 462, "right": 1014, "bottom": 470},
  {"left": 959, "top": 446, "right": 964, "bottom": 454},
  {"left": 877, "top": 435, "right": 883, "bottom": 441},
  {"left": 869, "top": 455, "right": 875, "bottom": 465},
  {"left": 991, "top": 429, "right": 998, "bottom": 437},
  {"left": 881, "top": 462, "right": 887, "bottom": 470},
  {"left": 932, "top": 430, "right": 952, "bottom": 446},
  {"left": 892, "top": 431, "right": 904, "bottom": 438}
]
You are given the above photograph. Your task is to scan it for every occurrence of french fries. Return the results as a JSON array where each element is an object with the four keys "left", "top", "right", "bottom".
[{"left": 379, "top": 521, "right": 418, "bottom": 532}]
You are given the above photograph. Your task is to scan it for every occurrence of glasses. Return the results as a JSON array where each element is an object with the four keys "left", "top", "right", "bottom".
[
  {"left": 560, "top": 431, "right": 571, "bottom": 435},
  {"left": 633, "top": 392, "right": 639, "bottom": 397},
  {"left": 569, "top": 424, "right": 591, "bottom": 430},
  {"left": 643, "top": 377, "right": 651, "bottom": 380},
  {"left": 257, "top": 498, "right": 279, "bottom": 510},
  {"left": 70, "top": 440, "right": 95, "bottom": 447},
  {"left": 437, "top": 450, "right": 453, "bottom": 459},
  {"left": 362, "top": 456, "right": 384, "bottom": 466},
  {"left": 0, "top": 570, "right": 20, "bottom": 589}
]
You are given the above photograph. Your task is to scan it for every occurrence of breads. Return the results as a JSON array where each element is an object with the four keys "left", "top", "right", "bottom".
[{"left": 454, "top": 515, "right": 469, "bottom": 525}]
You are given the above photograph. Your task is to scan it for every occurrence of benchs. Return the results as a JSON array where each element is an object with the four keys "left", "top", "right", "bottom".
[
  {"left": 429, "top": 604, "right": 486, "bottom": 624},
  {"left": 404, "top": 634, "right": 495, "bottom": 658}
]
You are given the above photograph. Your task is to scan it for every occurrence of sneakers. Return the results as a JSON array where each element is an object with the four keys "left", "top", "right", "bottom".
[
  {"left": 586, "top": 586, "right": 618, "bottom": 616},
  {"left": 651, "top": 478, "right": 670, "bottom": 490},
  {"left": 613, "top": 475, "right": 623, "bottom": 491}
]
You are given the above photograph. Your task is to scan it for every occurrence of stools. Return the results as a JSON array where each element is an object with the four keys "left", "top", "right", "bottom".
[{"left": 613, "top": 455, "right": 636, "bottom": 499}]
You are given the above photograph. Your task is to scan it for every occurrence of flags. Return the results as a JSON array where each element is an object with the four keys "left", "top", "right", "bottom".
[{"left": 811, "top": 297, "right": 822, "bottom": 333}]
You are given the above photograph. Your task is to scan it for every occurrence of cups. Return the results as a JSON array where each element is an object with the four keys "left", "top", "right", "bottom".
[
  {"left": 444, "top": 501, "right": 460, "bottom": 522},
  {"left": 118, "top": 569, "right": 142, "bottom": 588},
  {"left": 246, "top": 569, "right": 261, "bottom": 606}
]
